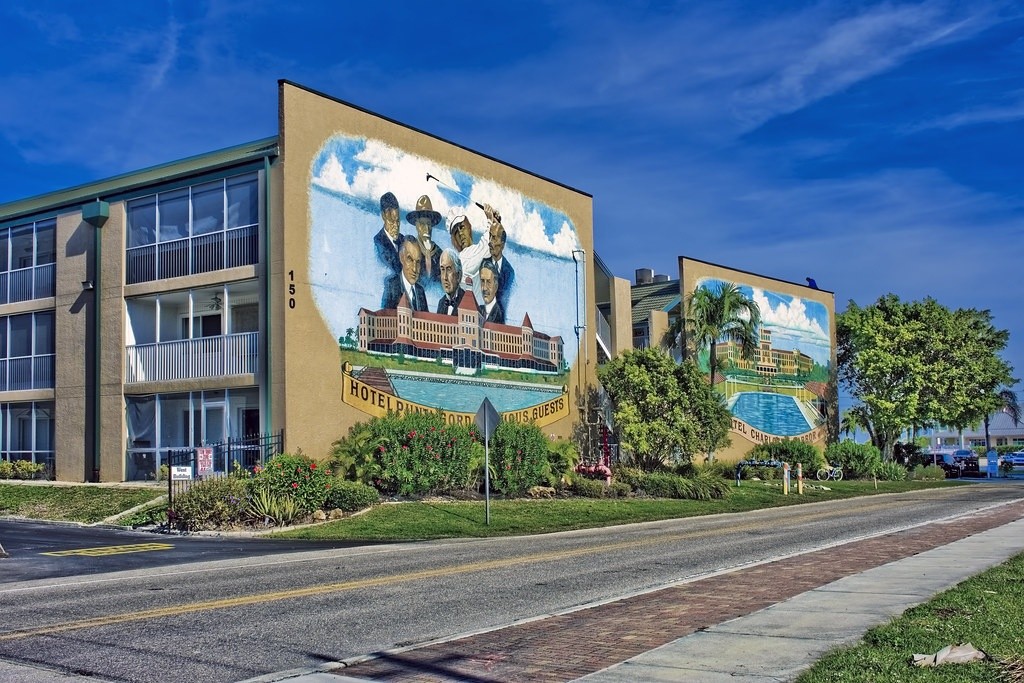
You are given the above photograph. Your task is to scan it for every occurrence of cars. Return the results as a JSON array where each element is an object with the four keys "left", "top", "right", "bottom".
[
  {"left": 950, "top": 449, "right": 979, "bottom": 467},
  {"left": 908, "top": 454, "right": 962, "bottom": 479}
]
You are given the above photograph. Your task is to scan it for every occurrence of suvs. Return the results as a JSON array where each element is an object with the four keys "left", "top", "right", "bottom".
[{"left": 1001, "top": 449, "right": 1024, "bottom": 465}]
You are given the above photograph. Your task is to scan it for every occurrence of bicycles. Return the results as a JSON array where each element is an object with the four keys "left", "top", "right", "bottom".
[{"left": 817, "top": 461, "right": 844, "bottom": 482}]
